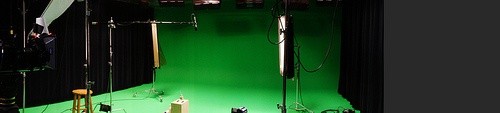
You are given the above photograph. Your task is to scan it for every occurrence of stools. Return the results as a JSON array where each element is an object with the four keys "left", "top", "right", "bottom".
[{"left": 72, "top": 89, "right": 93, "bottom": 113}]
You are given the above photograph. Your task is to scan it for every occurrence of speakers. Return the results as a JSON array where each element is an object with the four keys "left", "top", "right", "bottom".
[{"left": 278, "top": 15, "right": 294, "bottom": 79}]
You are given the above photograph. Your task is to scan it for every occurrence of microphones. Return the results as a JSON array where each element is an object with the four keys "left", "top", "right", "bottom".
[{"left": 190, "top": 13, "right": 198, "bottom": 30}]
